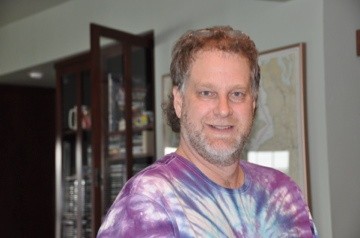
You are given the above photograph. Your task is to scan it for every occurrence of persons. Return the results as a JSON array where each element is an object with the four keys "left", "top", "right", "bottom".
[{"left": 95, "top": 25, "right": 319, "bottom": 238}]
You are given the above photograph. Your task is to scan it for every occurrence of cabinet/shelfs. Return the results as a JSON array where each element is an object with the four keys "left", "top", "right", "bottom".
[{"left": 54, "top": 22, "right": 156, "bottom": 238}]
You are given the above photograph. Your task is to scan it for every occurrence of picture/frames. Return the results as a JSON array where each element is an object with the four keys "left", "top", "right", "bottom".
[{"left": 161, "top": 41, "right": 313, "bottom": 219}]
